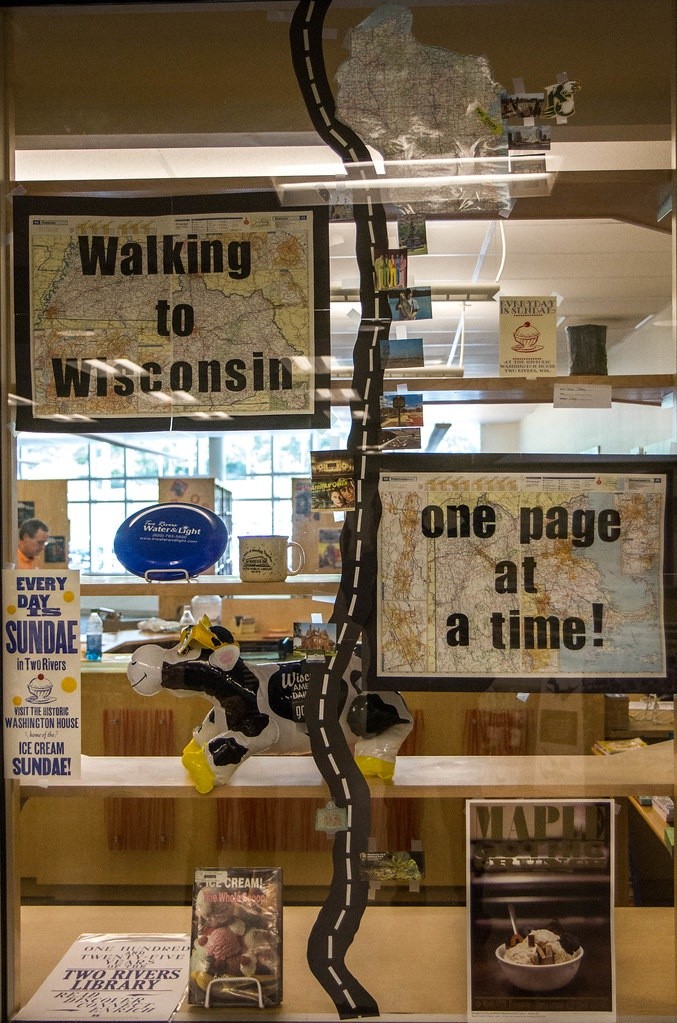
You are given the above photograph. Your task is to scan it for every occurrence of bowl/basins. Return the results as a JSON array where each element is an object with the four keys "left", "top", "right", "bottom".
[{"left": 495, "top": 943, "right": 583, "bottom": 991}]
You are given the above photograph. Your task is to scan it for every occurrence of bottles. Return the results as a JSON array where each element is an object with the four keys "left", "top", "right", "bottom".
[
  {"left": 179, "top": 606, "right": 195, "bottom": 633},
  {"left": 86, "top": 608, "right": 103, "bottom": 661}
]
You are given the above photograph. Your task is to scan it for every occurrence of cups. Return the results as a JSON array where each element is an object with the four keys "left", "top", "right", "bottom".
[
  {"left": 237, "top": 535, "right": 306, "bottom": 582},
  {"left": 564, "top": 323, "right": 609, "bottom": 376}
]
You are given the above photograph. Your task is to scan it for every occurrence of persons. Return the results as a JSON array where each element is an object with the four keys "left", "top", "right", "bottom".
[
  {"left": 375, "top": 253, "right": 406, "bottom": 291},
  {"left": 328, "top": 477, "right": 355, "bottom": 508},
  {"left": 18, "top": 518, "right": 52, "bottom": 570},
  {"left": 395, "top": 288, "right": 420, "bottom": 320}
]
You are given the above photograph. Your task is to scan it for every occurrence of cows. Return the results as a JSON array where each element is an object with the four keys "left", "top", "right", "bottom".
[{"left": 127, "top": 622, "right": 415, "bottom": 795}]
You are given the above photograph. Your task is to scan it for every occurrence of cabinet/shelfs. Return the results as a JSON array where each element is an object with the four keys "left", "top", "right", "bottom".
[{"left": 0, "top": 1, "right": 677, "bottom": 1022}]
